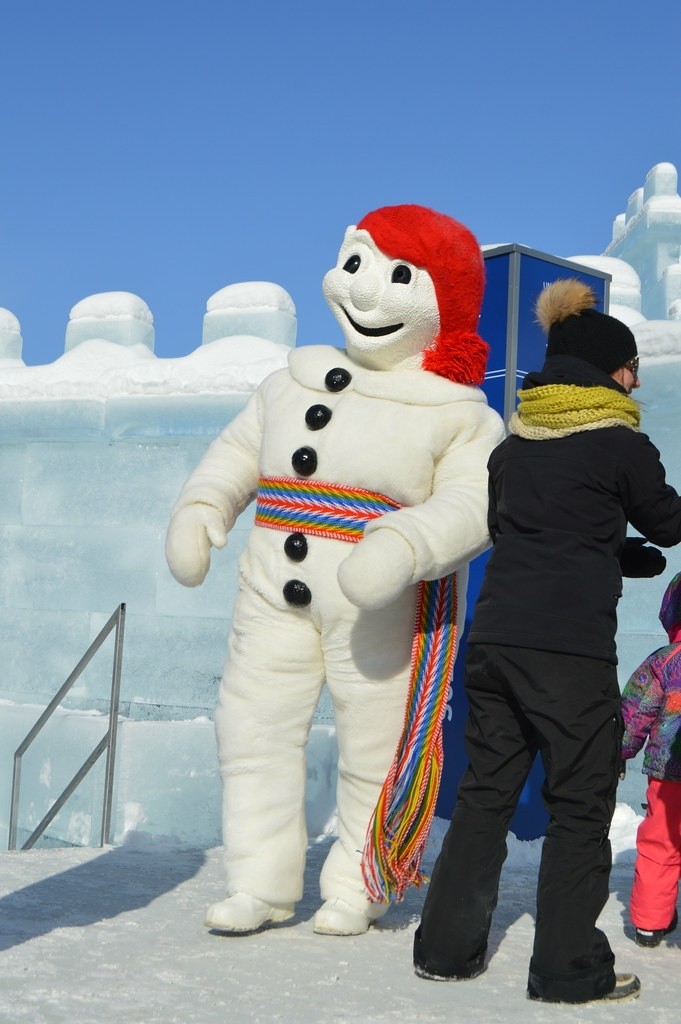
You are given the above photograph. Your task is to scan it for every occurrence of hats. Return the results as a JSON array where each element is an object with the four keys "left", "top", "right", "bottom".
[{"left": 535, "top": 279, "right": 638, "bottom": 375}]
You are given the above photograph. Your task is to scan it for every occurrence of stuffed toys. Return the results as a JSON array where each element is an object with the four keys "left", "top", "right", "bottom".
[{"left": 164, "top": 204, "right": 509, "bottom": 937}]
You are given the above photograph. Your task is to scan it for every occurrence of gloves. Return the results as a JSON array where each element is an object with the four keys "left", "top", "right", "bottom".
[{"left": 624, "top": 537, "right": 667, "bottom": 579}]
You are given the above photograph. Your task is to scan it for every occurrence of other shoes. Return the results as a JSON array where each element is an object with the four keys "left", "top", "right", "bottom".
[
  {"left": 585, "top": 972, "right": 642, "bottom": 1003},
  {"left": 635, "top": 907, "right": 678, "bottom": 948}
]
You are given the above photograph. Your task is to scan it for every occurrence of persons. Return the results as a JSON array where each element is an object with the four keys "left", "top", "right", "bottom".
[
  {"left": 621, "top": 573, "right": 680, "bottom": 949},
  {"left": 414, "top": 277, "right": 680, "bottom": 1004}
]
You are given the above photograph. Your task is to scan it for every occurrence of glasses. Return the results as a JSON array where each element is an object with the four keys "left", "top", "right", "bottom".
[{"left": 626, "top": 357, "right": 639, "bottom": 380}]
